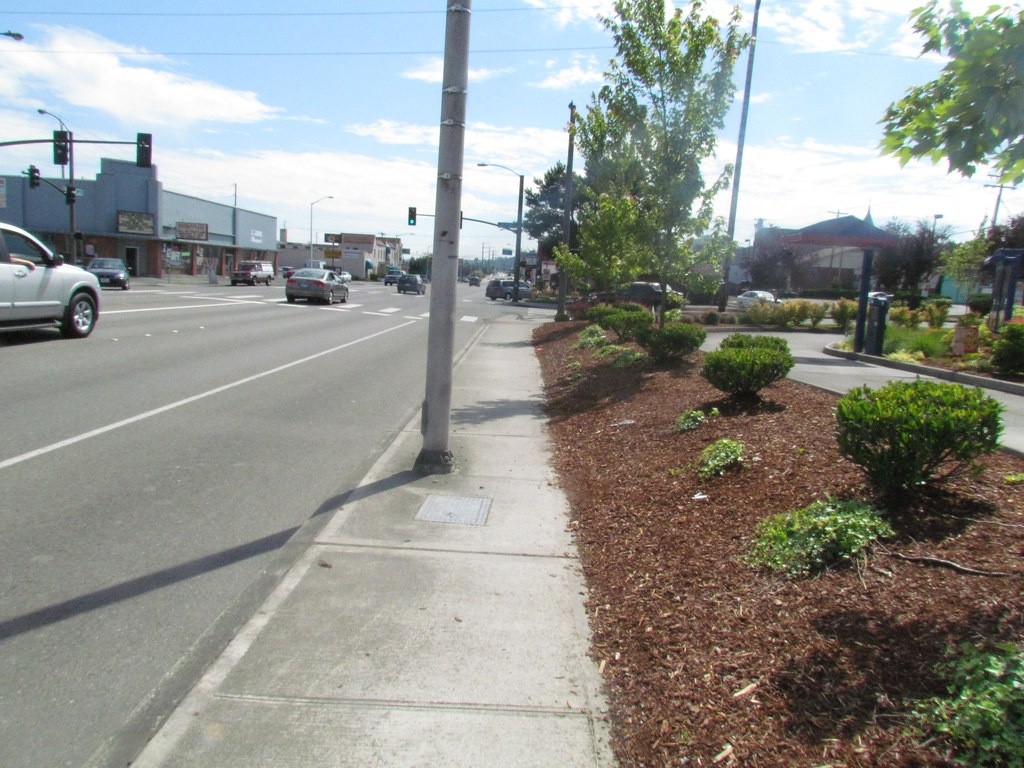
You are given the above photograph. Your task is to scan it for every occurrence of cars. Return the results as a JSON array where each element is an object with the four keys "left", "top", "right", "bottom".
[
  {"left": 734, "top": 289, "right": 782, "bottom": 309},
  {"left": 459, "top": 277, "right": 469, "bottom": 283},
  {"left": 420, "top": 274, "right": 430, "bottom": 284},
  {"left": 397, "top": 273, "right": 426, "bottom": 295},
  {"left": 854, "top": 289, "right": 895, "bottom": 307},
  {"left": 285, "top": 258, "right": 327, "bottom": 279},
  {"left": 469, "top": 277, "right": 481, "bottom": 287},
  {"left": 587, "top": 280, "right": 685, "bottom": 311},
  {"left": 0, "top": 221, "right": 101, "bottom": 338},
  {"left": 486, "top": 279, "right": 531, "bottom": 301},
  {"left": 285, "top": 268, "right": 350, "bottom": 304},
  {"left": 85, "top": 258, "right": 132, "bottom": 291},
  {"left": 339, "top": 271, "right": 352, "bottom": 282}
]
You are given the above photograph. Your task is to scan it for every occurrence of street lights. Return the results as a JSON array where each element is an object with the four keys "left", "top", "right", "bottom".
[
  {"left": 394, "top": 233, "right": 416, "bottom": 269},
  {"left": 310, "top": 196, "right": 334, "bottom": 269},
  {"left": 477, "top": 162, "right": 525, "bottom": 298},
  {"left": 932, "top": 215, "right": 943, "bottom": 233},
  {"left": 744, "top": 239, "right": 750, "bottom": 283},
  {"left": 38, "top": 110, "right": 76, "bottom": 261}
]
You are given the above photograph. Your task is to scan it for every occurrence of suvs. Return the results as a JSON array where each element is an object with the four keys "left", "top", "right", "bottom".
[
  {"left": 496, "top": 277, "right": 541, "bottom": 297},
  {"left": 231, "top": 259, "right": 274, "bottom": 286},
  {"left": 384, "top": 270, "right": 407, "bottom": 286}
]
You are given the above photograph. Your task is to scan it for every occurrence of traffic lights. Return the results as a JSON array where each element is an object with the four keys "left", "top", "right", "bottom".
[
  {"left": 66, "top": 184, "right": 76, "bottom": 205},
  {"left": 29, "top": 165, "right": 40, "bottom": 190},
  {"left": 408, "top": 207, "right": 416, "bottom": 225},
  {"left": 74, "top": 231, "right": 82, "bottom": 240}
]
